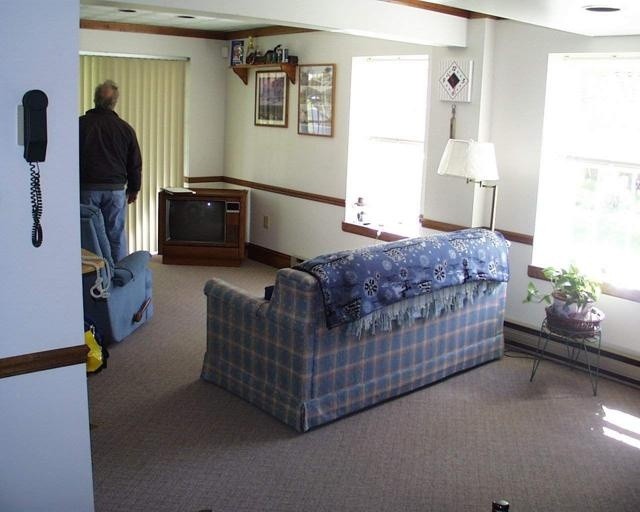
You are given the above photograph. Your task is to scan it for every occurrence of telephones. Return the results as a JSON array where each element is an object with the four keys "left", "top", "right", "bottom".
[{"left": 20, "top": 87, "right": 49, "bottom": 164}]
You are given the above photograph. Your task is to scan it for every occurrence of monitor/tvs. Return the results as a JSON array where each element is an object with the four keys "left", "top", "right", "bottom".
[{"left": 157, "top": 187, "right": 249, "bottom": 268}]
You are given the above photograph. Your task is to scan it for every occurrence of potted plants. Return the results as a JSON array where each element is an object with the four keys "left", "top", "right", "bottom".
[{"left": 529, "top": 262, "right": 608, "bottom": 335}]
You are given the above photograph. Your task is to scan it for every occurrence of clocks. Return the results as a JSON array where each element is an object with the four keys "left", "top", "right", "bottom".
[{"left": 432, "top": 58, "right": 475, "bottom": 139}]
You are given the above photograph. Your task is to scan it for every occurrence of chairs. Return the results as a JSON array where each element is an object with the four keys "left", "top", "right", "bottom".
[{"left": 80, "top": 205, "right": 155, "bottom": 345}]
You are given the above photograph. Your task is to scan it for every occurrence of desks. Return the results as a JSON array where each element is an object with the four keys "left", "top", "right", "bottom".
[
  {"left": 80, "top": 247, "right": 106, "bottom": 274},
  {"left": 530, "top": 316, "right": 601, "bottom": 394}
]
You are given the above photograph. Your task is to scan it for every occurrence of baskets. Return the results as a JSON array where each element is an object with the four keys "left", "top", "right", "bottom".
[{"left": 545, "top": 305, "right": 605, "bottom": 339}]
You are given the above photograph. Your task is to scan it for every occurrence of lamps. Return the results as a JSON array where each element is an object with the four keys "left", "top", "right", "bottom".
[{"left": 436, "top": 138, "right": 499, "bottom": 234}]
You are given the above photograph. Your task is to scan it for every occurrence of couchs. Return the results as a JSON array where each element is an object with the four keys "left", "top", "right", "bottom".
[{"left": 200, "top": 226, "right": 512, "bottom": 435}]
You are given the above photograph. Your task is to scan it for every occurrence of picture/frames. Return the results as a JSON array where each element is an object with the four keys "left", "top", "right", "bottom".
[
  {"left": 297, "top": 64, "right": 337, "bottom": 137},
  {"left": 253, "top": 69, "right": 286, "bottom": 129}
]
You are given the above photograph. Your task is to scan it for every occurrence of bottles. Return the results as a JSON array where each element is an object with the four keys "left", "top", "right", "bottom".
[{"left": 244, "top": 34, "right": 256, "bottom": 64}]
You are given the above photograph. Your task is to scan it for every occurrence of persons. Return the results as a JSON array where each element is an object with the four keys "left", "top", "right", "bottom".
[
  {"left": 79, "top": 79, "right": 142, "bottom": 282},
  {"left": 233, "top": 45, "right": 243, "bottom": 61}
]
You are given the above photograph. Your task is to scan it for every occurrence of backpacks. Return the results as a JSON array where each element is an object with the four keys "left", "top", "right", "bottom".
[{"left": 85, "top": 315, "right": 110, "bottom": 375}]
